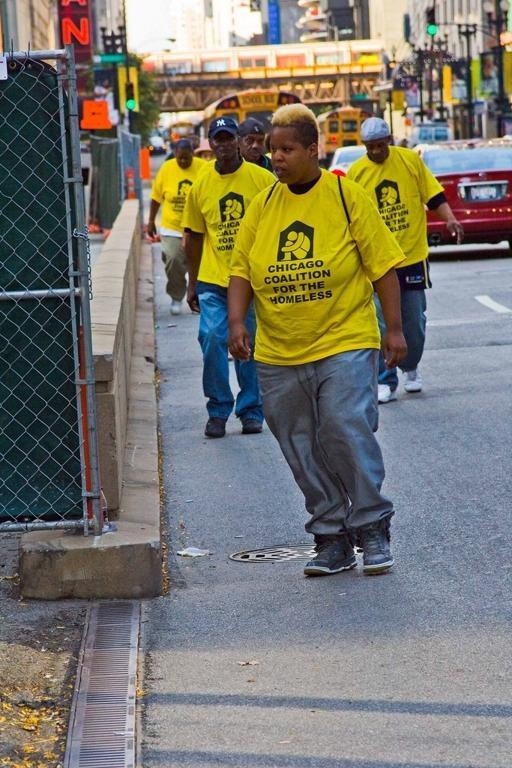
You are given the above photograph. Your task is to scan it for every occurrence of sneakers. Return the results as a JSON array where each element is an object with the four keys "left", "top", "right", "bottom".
[
  {"left": 376, "top": 384, "right": 399, "bottom": 403},
  {"left": 359, "top": 523, "right": 395, "bottom": 574},
  {"left": 204, "top": 415, "right": 229, "bottom": 437},
  {"left": 303, "top": 538, "right": 358, "bottom": 577},
  {"left": 404, "top": 367, "right": 424, "bottom": 392},
  {"left": 170, "top": 299, "right": 184, "bottom": 315},
  {"left": 241, "top": 419, "right": 264, "bottom": 434}
]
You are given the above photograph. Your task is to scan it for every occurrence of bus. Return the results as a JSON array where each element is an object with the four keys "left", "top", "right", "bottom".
[{"left": 166, "top": 85, "right": 381, "bottom": 179}]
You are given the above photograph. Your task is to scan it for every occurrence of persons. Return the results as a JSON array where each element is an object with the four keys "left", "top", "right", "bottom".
[
  {"left": 235, "top": 114, "right": 277, "bottom": 174},
  {"left": 182, "top": 116, "right": 283, "bottom": 438},
  {"left": 192, "top": 136, "right": 217, "bottom": 162},
  {"left": 345, "top": 116, "right": 467, "bottom": 406},
  {"left": 227, "top": 101, "right": 397, "bottom": 575},
  {"left": 148, "top": 137, "right": 211, "bottom": 318},
  {"left": 483, "top": 53, "right": 498, "bottom": 94}
]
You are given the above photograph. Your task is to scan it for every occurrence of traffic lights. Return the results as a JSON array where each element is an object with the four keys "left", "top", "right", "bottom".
[
  {"left": 125, "top": 82, "right": 135, "bottom": 110},
  {"left": 387, "top": 60, "right": 397, "bottom": 81},
  {"left": 424, "top": 7, "right": 437, "bottom": 36}
]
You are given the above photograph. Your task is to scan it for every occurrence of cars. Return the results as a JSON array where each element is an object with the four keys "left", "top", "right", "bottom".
[
  {"left": 324, "top": 122, "right": 511, "bottom": 251},
  {"left": 145, "top": 135, "right": 166, "bottom": 155}
]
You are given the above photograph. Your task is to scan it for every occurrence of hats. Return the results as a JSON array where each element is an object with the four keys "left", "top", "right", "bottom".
[
  {"left": 237, "top": 116, "right": 266, "bottom": 137},
  {"left": 207, "top": 116, "right": 238, "bottom": 138},
  {"left": 360, "top": 116, "right": 390, "bottom": 143}
]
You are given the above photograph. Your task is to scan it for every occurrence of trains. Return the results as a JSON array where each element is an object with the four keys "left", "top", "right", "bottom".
[{"left": 138, "top": 40, "right": 397, "bottom": 90}]
